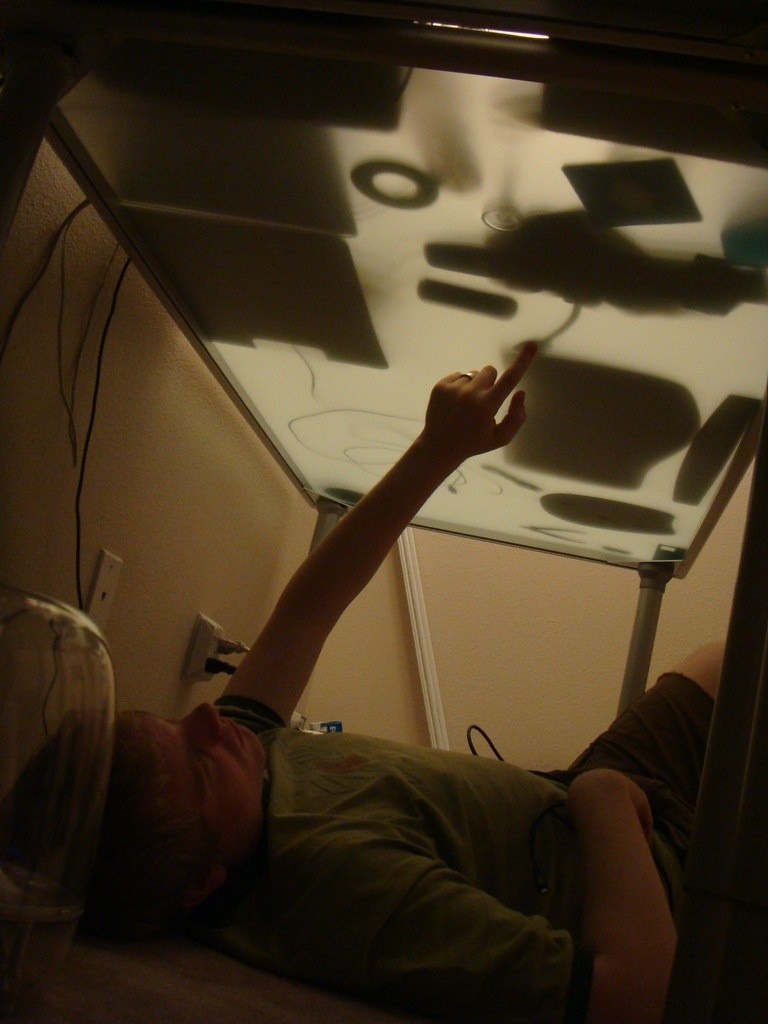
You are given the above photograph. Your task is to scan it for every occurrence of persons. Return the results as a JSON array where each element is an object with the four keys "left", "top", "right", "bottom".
[{"left": 0, "top": 341, "right": 725, "bottom": 1024}]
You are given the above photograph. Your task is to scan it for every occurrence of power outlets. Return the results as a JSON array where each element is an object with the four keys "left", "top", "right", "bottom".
[{"left": 182, "top": 613, "right": 227, "bottom": 681}]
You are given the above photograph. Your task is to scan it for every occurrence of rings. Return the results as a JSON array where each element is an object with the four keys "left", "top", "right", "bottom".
[{"left": 458, "top": 372, "right": 473, "bottom": 378}]
